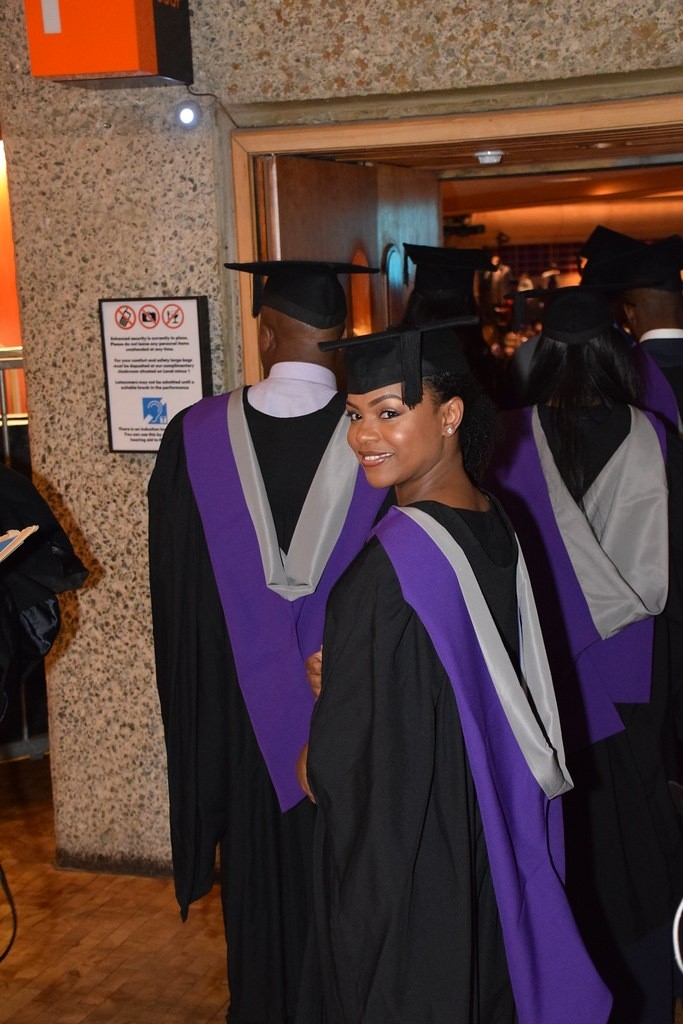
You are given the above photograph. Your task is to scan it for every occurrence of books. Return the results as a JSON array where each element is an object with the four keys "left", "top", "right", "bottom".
[{"left": 0, "top": 523, "right": 40, "bottom": 565}]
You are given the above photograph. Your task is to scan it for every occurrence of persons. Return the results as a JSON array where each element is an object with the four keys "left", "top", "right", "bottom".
[
  {"left": 0, "top": 439, "right": 90, "bottom": 724},
  {"left": 294, "top": 310, "right": 575, "bottom": 1024},
  {"left": 146, "top": 261, "right": 397, "bottom": 1024},
  {"left": 400, "top": 225, "right": 683, "bottom": 1024}
]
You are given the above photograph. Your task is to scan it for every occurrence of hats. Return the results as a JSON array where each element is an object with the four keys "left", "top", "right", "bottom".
[
  {"left": 402, "top": 243, "right": 510, "bottom": 295},
  {"left": 224, "top": 261, "right": 381, "bottom": 330},
  {"left": 317, "top": 314, "right": 504, "bottom": 409},
  {"left": 589, "top": 234, "right": 683, "bottom": 291},
  {"left": 575, "top": 227, "right": 650, "bottom": 276},
  {"left": 503, "top": 287, "right": 639, "bottom": 345}
]
